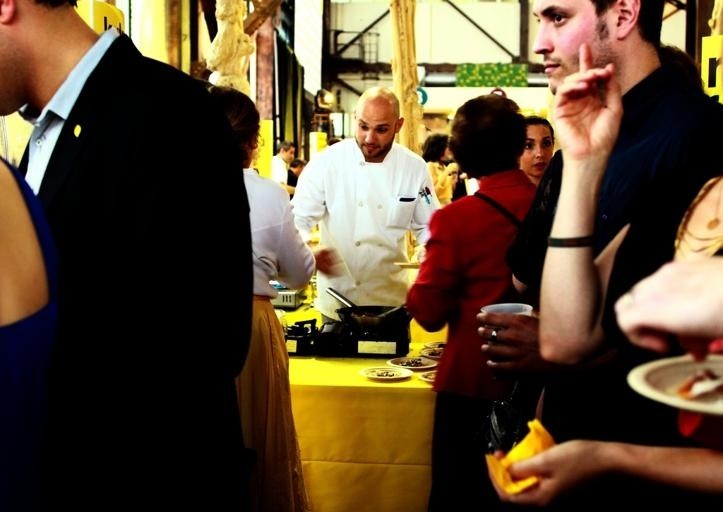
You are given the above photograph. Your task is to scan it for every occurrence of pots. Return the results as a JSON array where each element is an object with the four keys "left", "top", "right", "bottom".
[{"left": 326, "top": 286, "right": 412, "bottom": 331}]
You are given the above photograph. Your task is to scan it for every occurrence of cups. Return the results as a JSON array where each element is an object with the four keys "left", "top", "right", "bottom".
[{"left": 482, "top": 303, "right": 534, "bottom": 316}]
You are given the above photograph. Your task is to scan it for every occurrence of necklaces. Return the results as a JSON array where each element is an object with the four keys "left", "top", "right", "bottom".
[{"left": 673, "top": 179, "right": 721, "bottom": 258}]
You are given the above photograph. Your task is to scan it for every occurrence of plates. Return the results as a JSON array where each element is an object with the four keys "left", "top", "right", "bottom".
[
  {"left": 422, "top": 348, "right": 444, "bottom": 359},
  {"left": 418, "top": 371, "right": 436, "bottom": 382},
  {"left": 425, "top": 341, "right": 446, "bottom": 349},
  {"left": 626, "top": 355, "right": 723, "bottom": 416},
  {"left": 385, "top": 356, "right": 438, "bottom": 369},
  {"left": 360, "top": 367, "right": 414, "bottom": 381}
]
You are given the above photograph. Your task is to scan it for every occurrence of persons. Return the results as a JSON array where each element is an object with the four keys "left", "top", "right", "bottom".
[
  {"left": 289, "top": 86, "right": 446, "bottom": 345},
  {"left": 421, "top": 134, "right": 458, "bottom": 208},
  {"left": 407, "top": 95, "right": 539, "bottom": 512},
  {"left": 0, "top": 0, "right": 255, "bottom": 511},
  {"left": 613, "top": 256, "right": 723, "bottom": 352},
  {"left": 287, "top": 159, "right": 305, "bottom": 199},
  {"left": 217, "top": 86, "right": 316, "bottom": 511},
  {"left": 273, "top": 142, "right": 296, "bottom": 196},
  {"left": 0, "top": 158, "right": 76, "bottom": 511},
  {"left": 520, "top": 115, "right": 555, "bottom": 184}
]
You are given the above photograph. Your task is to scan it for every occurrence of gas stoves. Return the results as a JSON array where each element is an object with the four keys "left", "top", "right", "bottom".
[{"left": 282, "top": 319, "right": 410, "bottom": 358}]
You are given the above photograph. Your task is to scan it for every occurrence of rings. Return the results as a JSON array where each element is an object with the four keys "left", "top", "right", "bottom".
[{"left": 492, "top": 327, "right": 501, "bottom": 344}]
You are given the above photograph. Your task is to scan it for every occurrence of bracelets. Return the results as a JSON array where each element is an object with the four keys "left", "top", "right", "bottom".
[{"left": 547, "top": 235, "right": 596, "bottom": 248}]
